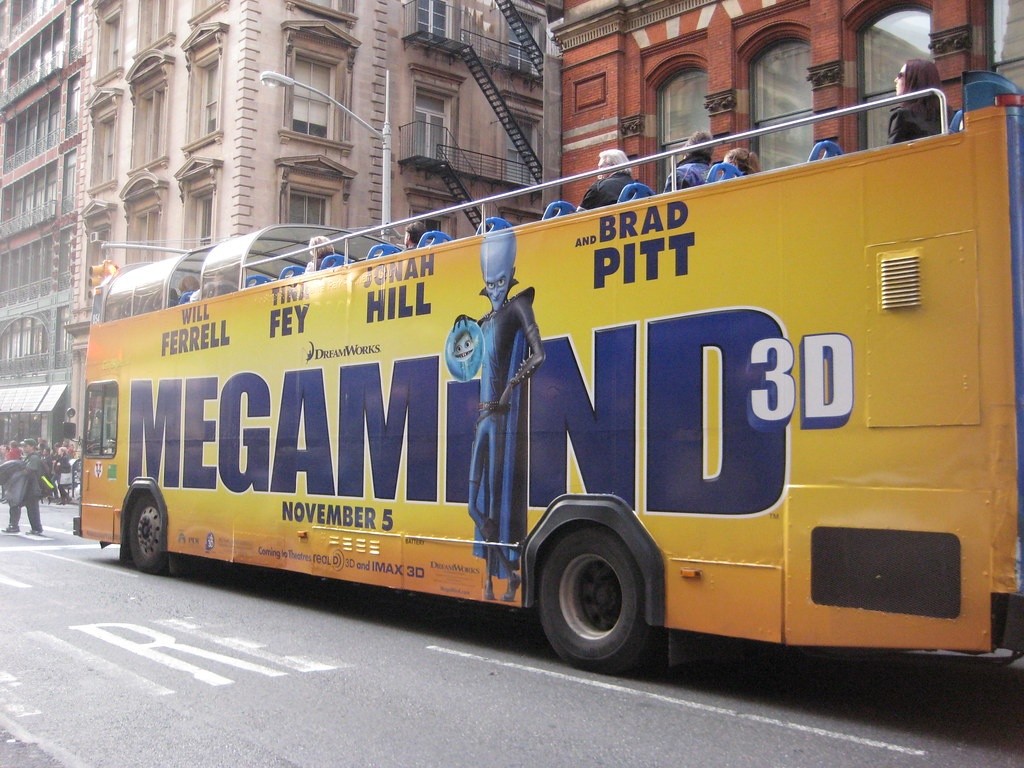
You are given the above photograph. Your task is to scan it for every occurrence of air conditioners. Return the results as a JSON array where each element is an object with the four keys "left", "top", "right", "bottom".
[{"left": 90, "top": 231, "right": 106, "bottom": 244}]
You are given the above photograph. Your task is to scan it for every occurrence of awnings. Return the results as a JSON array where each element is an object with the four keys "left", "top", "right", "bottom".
[{"left": 0, "top": 384, "right": 68, "bottom": 412}]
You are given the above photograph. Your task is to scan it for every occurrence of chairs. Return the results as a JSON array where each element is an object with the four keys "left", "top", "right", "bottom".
[
  {"left": 366, "top": 244, "right": 400, "bottom": 260},
  {"left": 207, "top": 284, "right": 238, "bottom": 298},
  {"left": 278, "top": 265, "right": 306, "bottom": 280},
  {"left": 617, "top": 183, "right": 655, "bottom": 204},
  {"left": 807, "top": 140, "right": 844, "bottom": 162},
  {"left": 705, "top": 162, "right": 744, "bottom": 184},
  {"left": 416, "top": 230, "right": 453, "bottom": 248},
  {"left": 947, "top": 110, "right": 965, "bottom": 133},
  {"left": 475, "top": 216, "right": 512, "bottom": 235},
  {"left": 178, "top": 290, "right": 197, "bottom": 305},
  {"left": 241, "top": 275, "right": 272, "bottom": 289},
  {"left": 319, "top": 254, "right": 354, "bottom": 270},
  {"left": 542, "top": 201, "right": 578, "bottom": 221}
]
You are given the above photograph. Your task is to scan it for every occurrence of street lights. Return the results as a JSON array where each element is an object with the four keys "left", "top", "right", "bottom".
[{"left": 256, "top": 69, "right": 394, "bottom": 243}]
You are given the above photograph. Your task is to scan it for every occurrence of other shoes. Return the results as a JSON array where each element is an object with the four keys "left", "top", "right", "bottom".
[
  {"left": 1, "top": 526, "right": 19, "bottom": 532},
  {"left": 26, "top": 529, "right": 41, "bottom": 534}
]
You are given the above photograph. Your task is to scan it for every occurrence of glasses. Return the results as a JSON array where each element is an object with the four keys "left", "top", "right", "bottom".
[{"left": 897, "top": 72, "right": 906, "bottom": 79}]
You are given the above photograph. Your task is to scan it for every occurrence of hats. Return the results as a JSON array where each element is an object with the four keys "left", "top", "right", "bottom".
[{"left": 20, "top": 438, "right": 37, "bottom": 446}]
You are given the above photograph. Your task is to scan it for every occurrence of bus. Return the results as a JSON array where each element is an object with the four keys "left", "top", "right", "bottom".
[{"left": 63, "top": 71, "right": 1024, "bottom": 674}]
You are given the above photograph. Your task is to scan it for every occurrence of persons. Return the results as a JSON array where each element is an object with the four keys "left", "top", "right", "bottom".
[
  {"left": 723, "top": 148, "right": 760, "bottom": 176},
  {"left": 401, "top": 221, "right": 428, "bottom": 253},
  {"left": 304, "top": 235, "right": 337, "bottom": 273},
  {"left": 577, "top": 149, "right": 637, "bottom": 211},
  {"left": 885, "top": 59, "right": 956, "bottom": 145},
  {"left": 151, "top": 266, "right": 240, "bottom": 311},
  {"left": 0, "top": 437, "right": 75, "bottom": 535},
  {"left": 663, "top": 131, "right": 713, "bottom": 193}
]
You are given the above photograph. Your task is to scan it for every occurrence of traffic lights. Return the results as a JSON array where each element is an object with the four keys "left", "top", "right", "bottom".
[
  {"left": 109, "top": 264, "right": 119, "bottom": 274},
  {"left": 89, "top": 265, "right": 103, "bottom": 299}
]
